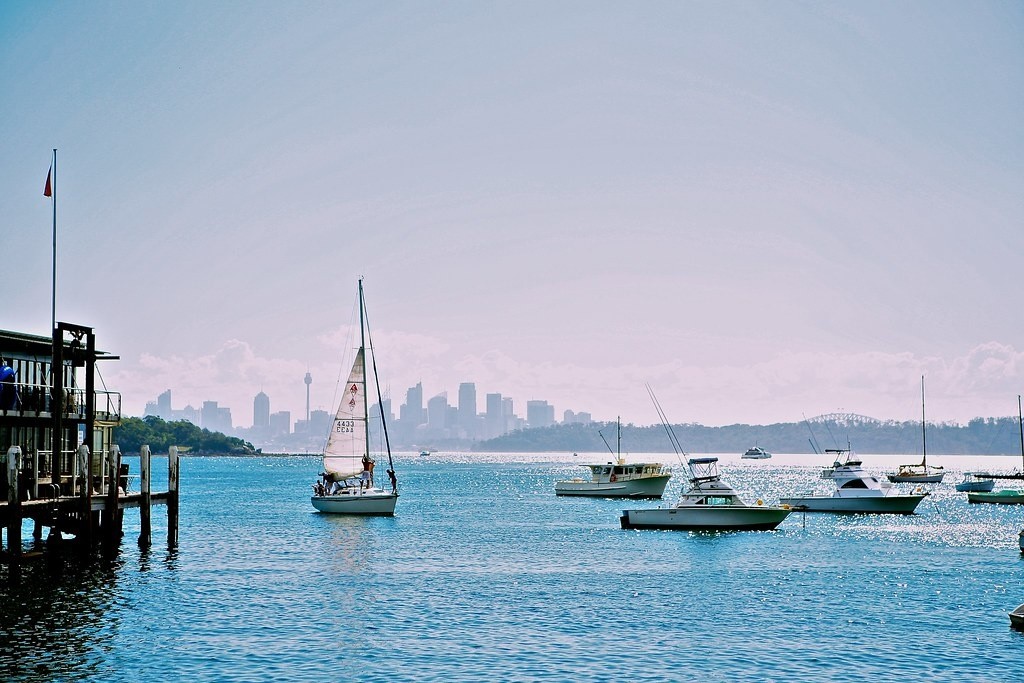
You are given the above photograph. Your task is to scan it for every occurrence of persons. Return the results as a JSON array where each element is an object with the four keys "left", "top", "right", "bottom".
[{"left": 312, "top": 453, "right": 376, "bottom": 497}]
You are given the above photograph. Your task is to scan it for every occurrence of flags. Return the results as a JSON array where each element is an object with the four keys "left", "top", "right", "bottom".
[{"left": 44, "top": 156, "right": 52, "bottom": 197}]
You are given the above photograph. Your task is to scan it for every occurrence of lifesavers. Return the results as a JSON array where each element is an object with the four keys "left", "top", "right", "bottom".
[{"left": 609, "top": 473, "right": 616, "bottom": 482}]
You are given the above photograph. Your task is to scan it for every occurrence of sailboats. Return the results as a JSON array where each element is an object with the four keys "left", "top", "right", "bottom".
[
  {"left": 972, "top": 393, "right": 1024, "bottom": 480},
  {"left": 310, "top": 277, "right": 400, "bottom": 518},
  {"left": 886, "top": 373, "right": 944, "bottom": 485}
]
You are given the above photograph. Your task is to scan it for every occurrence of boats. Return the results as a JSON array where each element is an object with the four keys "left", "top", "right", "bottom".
[
  {"left": 740, "top": 442, "right": 772, "bottom": 460},
  {"left": 956, "top": 471, "right": 995, "bottom": 492},
  {"left": 821, "top": 446, "right": 863, "bottom": 478},
  {"left": 619, "top": 382, "right": 796, "bottom": 532},
  {"left": 554, "top": 415, "right": 671, "bottom": 500},
  {"left": 1008, "top": 603, "right": 1024, "bottom": 625},
  {"left": 774, "top": 471, "right": 930, "bottom": 516},
  {"left": 969, "top": 486, "right": 1024, "bottom": 507}
]
